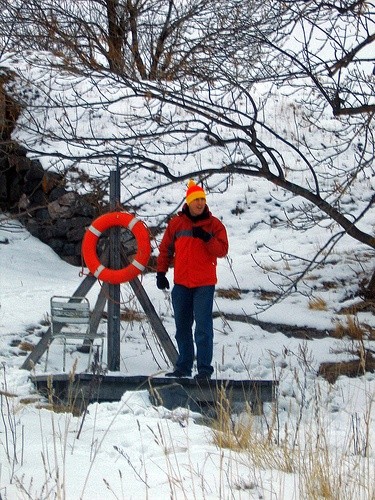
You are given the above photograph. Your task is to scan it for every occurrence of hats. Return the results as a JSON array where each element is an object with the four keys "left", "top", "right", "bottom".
[{"left": 187, "top": 180, "right": 206, "bottom": 206}]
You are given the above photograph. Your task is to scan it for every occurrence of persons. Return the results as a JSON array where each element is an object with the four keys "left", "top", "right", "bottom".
[{"left": 156, "top": 181, "right": 230, "bottom": 380}]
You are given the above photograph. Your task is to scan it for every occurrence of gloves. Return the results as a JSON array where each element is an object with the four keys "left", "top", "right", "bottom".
[
  {"left": 192, "top": 226, "right": 211, "bottom": 242},
  {"left": 156, "top": 272, "right": 169, "bottom": 290}
]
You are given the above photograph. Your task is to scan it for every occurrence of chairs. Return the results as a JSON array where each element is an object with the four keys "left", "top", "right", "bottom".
[{"left": 44, "top": 296, "right": 104, "bottom": 372}]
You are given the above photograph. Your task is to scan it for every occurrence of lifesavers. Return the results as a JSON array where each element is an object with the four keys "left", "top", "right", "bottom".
[{"left": 83, "top": 210, "right": 152, "bottom": 283}]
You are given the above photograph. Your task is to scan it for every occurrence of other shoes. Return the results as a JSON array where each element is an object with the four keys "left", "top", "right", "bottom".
[
  {"left": 194, "top": 371, "right": 211, "bottom": 380},
  {"left": 165, "top": 370, "right": 191, "bottom": 377}
]
